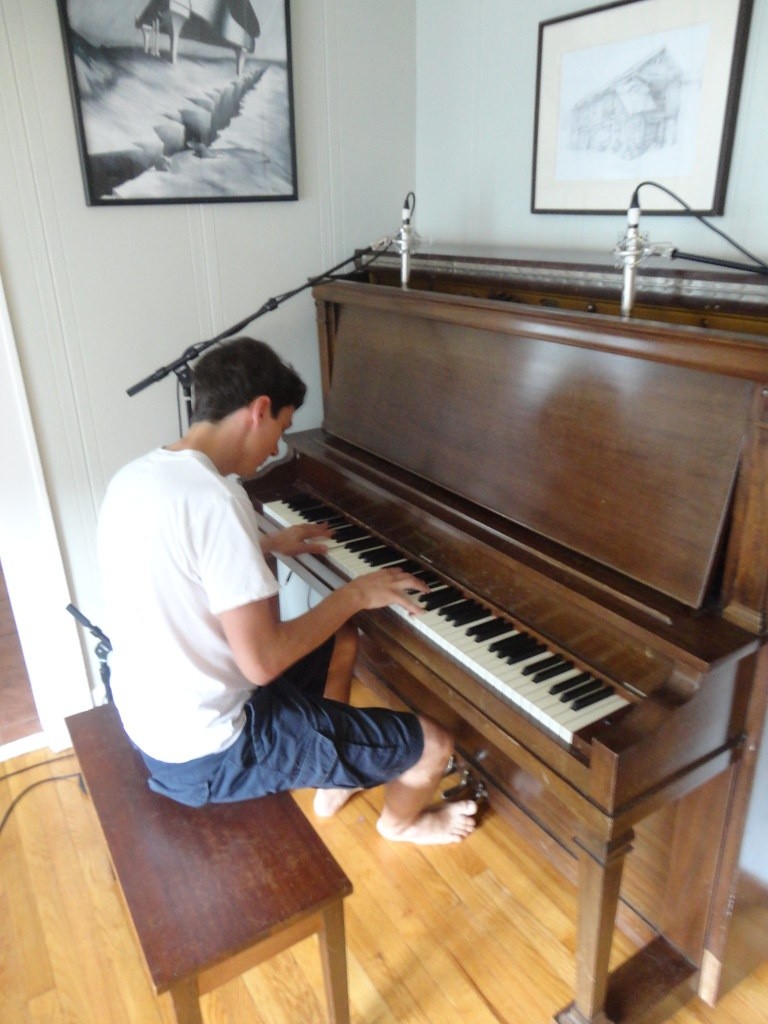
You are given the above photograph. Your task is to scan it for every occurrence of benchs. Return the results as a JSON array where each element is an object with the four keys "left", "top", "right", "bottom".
[{"left": 69, "top": 683, "right": 359, "bottom": 1024}]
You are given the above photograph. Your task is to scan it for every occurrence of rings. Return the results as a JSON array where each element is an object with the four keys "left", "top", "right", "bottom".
[{"left": 302, "top": 525, "right": 306, "bottom": 528}]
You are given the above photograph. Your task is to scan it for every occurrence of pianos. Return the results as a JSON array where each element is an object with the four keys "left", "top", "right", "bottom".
[{"left": 238, "top": 243, "right": 768, "bottom": 1023}]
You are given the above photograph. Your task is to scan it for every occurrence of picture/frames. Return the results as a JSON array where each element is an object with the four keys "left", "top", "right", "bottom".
[
  {"left": 55, "top": 0, "right": 302, "bottom": 209},
  {"left": 525, "top": 0, "right": 753, "bottom": 222}
]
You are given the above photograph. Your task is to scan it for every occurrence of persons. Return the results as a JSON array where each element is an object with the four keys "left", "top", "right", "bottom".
[{"left": 94, "top": 337, "right": 478, "bottom": 848}]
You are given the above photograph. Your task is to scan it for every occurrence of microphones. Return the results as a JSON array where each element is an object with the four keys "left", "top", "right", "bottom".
[
  {"left": 621, "top": 191, "right": 642, "bottom": 316},
  {"left": 401, "top": 199, "right": 414, "bottom": 286}
]
